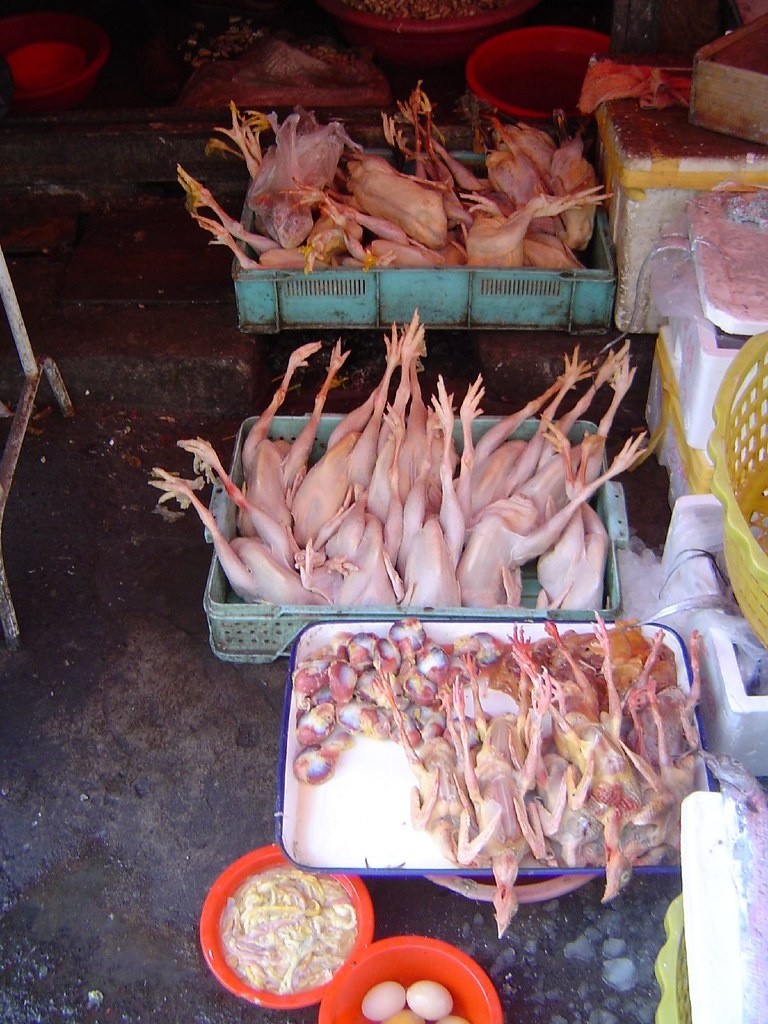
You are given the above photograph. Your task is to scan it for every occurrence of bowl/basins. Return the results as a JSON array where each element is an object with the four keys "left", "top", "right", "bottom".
[
  {"left": 316, "top": 935, "right": 503, "bottom": 1024},
  {"left": 323, "top": 1, "right": 542, "bottom": 70},
  {"left": 0, "top": 12, "right": 111, "bottom": 113},
  {"left": 199, "top": 846, "right": 376, "bottom": 1009},
  {"left": 6, "top": 42, "right": 88, "bottom": 94},
  {"left": 464, "top": 25, "right": 613, "bottom": 123}
]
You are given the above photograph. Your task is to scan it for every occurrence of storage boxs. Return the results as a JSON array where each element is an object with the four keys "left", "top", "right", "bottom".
[
  {"left": 648, "top": 492, "right": 767, "bottom": 780},
  {"left": 705, "top": 331, "right": 766, "bottom": 650},
  {"left": 316, "top": 1, "right": 536, "bottom": 67},
  {"left": 645, "top": 326, "right": 714, "bottom": 515},
  {"left": 590, "top": 52, "right": 767, "bottom": 336},
  {"left": 230, "top": 146, "right": 619, "bottom": 334},
  {"left": 0, "top": 12, "right": 112, "bottom": 115},
  {"left": 316, "top": 935, "right": 505, "bottom": 1024},
  {"left": 655, "top": 893, "right": 691, "bottom": 1023},
  {"left": 202, "top": 411, "right": 632, "bottom": 666},
  {"left": 680, "top": 788, "right": 768, "bottom": 1023},
  {"left": 667, "top": 238, "right": 767, "bottom": 453},
  {"left": 465, "top": 24, "right": 611, "bottom": 124},
  {"left": 688, "top": 11, "right": 767, "bottom": 147},
  {"left": 274, "top": 617, "right": 718, "bottom": 881},
  {"left": 199, "top": 844, "right": 375, "bottom": 1009},
  {"left": 423, "top": 875, "right": 603, "bottom": 903}
]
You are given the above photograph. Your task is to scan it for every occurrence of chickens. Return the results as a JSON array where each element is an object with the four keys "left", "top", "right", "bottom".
[{"left": 148, "top": 77, "right": 701, "bottom": 938}]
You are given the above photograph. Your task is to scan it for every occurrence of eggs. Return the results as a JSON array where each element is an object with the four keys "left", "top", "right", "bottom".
[{"left": 362, "top": 980, "right": 471, "bottom": 1024}]
